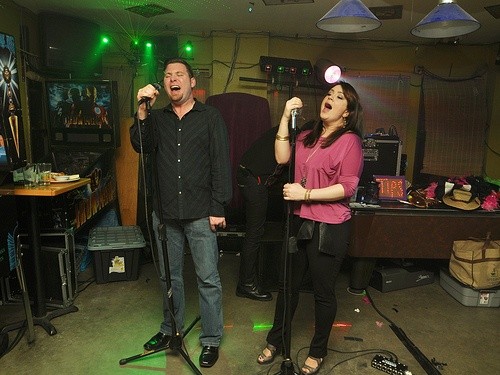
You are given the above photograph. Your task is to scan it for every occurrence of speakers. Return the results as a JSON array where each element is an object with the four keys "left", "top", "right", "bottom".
[{"left": 155, "top": 35, "right": 179, "bottom": 62}]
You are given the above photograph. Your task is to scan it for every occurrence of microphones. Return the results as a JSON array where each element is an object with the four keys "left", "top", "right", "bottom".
[
  {"left": 137, "top": 82, "right": 163, "bottom": 104},
  {"left": 290, "top": 107, "right": 299, "bottom": 130}
]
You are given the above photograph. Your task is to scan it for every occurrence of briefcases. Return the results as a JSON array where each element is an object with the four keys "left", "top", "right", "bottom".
[
  {"left": 370, "top": 265, "right": 434, "bottom": 292},
  {"left": 439, "top": 269, "right": 500, "bottom": 306}
]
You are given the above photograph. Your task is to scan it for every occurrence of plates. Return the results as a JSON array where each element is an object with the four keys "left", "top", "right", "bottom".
[{"left": 51, "top": 178, "right": 80, "bottom": 183}]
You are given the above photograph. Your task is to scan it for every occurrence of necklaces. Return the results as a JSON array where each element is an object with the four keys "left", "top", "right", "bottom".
[{"left": 301, "top": 140, "right": 324, "bottom": 188}]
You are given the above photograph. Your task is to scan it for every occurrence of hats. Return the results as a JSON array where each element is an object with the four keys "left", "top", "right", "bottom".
[{"left": 443, "top": 185, "right": 481, "bottom": 210}]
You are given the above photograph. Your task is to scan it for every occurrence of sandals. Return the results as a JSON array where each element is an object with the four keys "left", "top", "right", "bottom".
[
  {"left": 257, "top": 344, "right": 281, "bottom": 363},
  {"left": 299, "top": 356, "right": 324, "bottom": 375}
]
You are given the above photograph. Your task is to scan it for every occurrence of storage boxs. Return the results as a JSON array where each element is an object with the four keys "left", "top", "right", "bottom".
[
  {"left": 18, "top": 228, "right": 80, "bottom": 307},
  {"left": 439, "top": 265, "right": 500, "bottom": 308},
  {"left": 358, "top": 135, "right": 403, "bottom": 188},
  {"left": 87, "top": 225, "right": 147, "bottom": 285}
]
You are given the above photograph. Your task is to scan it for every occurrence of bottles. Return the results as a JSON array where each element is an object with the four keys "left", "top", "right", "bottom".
[{"left": 366, "top": 175, "right": 380, "bottom": 204}]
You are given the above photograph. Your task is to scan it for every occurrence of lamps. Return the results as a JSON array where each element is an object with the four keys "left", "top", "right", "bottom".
[
  {"left": 315, "top": 0, "right": 383, "bottom": 33},
  {"left": 409, "top": 0, "right": 481, "bottom": 38},
  {"left": 313, "top": 58, "right": 342, "bottom": 84}
]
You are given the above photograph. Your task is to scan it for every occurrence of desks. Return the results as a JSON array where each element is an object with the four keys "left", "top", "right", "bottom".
[
  {"left": 0, "top": 169, "right": 117, "bottom": 308},
  {"left": 0, "top": 177, "right": 92, "bottom": 336},
  {"left": 342, "top": 189, "right": 500, "bottom": 296}
]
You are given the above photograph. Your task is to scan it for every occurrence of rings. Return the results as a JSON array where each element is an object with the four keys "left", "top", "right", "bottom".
[{"left": 284, "top": 192, "right": 286, "bottom": 196}]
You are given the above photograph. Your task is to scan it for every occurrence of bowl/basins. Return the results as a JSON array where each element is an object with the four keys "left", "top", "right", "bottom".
[{"left": 54, "top": 176, "right": 69, "bottom": 181}]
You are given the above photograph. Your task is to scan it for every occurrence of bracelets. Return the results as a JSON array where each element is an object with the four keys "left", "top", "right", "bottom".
[
  {"left": 276, "top": 134, "right": 290, "bottom": 141},
  {"left": 304, "top": 189, "right": 313, "bottom": 203}
]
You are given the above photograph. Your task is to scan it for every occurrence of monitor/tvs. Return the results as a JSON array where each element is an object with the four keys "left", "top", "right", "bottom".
[{"left": 44, "top": 78, "right": 117, "bottom": 132}]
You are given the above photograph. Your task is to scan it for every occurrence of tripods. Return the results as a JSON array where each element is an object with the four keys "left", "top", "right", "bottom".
[{"left": 117, "top": 99, "right": 202, "bottom": 375}]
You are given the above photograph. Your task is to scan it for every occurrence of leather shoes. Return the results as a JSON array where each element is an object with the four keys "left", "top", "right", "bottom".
[
  {"left": 199, "top": 345, "right": 218, "bottom": 366},
  {"left": 143, "top": 333, "right": 172, "bottom": 350},
  {"left": 236, "top": 279, "right": 271, "bottom": 300}
]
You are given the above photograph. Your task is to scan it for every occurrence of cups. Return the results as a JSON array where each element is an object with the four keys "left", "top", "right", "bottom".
[
  {"left": 37, "top": 163, "right": 52, "bottom": 186},
  {"left": 356, "top": 188, "right": 366, "bottom": 204},
  {"left": 22, "top": 163, "right": 39, "bottom": 187}
]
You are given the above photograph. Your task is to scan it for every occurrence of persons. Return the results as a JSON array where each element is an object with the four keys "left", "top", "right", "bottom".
[
  {"left": 129, "top": 58, "right": 231, "bottom": 367},
  {"left": 256, "top": 82, "right": 364, "bottom": 375},
  {"left": 236, "top": 120, "right": 320, "bottom": 301}
]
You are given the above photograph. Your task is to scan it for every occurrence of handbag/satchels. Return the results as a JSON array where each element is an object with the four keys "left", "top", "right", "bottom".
[{"left": 449, "top": 232, "right": 500, "bottom": 290}]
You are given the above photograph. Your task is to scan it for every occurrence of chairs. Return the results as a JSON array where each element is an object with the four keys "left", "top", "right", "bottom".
[{"left": 205, "top": 92, "right": 274, "bottom": 255}]
